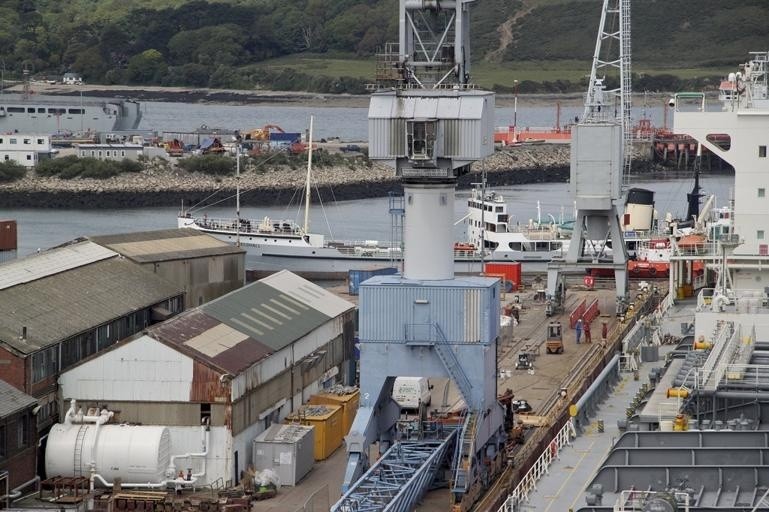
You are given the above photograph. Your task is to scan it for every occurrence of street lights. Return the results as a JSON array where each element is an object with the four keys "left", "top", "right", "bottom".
[{"left": 79, "top": 83, "right": 83, "bottom": 132}]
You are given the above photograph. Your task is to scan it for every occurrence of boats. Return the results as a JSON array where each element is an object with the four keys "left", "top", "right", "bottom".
[{"left": 0, "top": 93, "right": 143, "bottom": 132}]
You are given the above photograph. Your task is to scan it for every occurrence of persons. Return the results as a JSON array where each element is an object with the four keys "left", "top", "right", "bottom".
[
  {"left": 571, "top": 318, "right": 582, "bottom": 346},
  {"left": 583, "top": 319, "right": 591, "bottom": 342}
]
